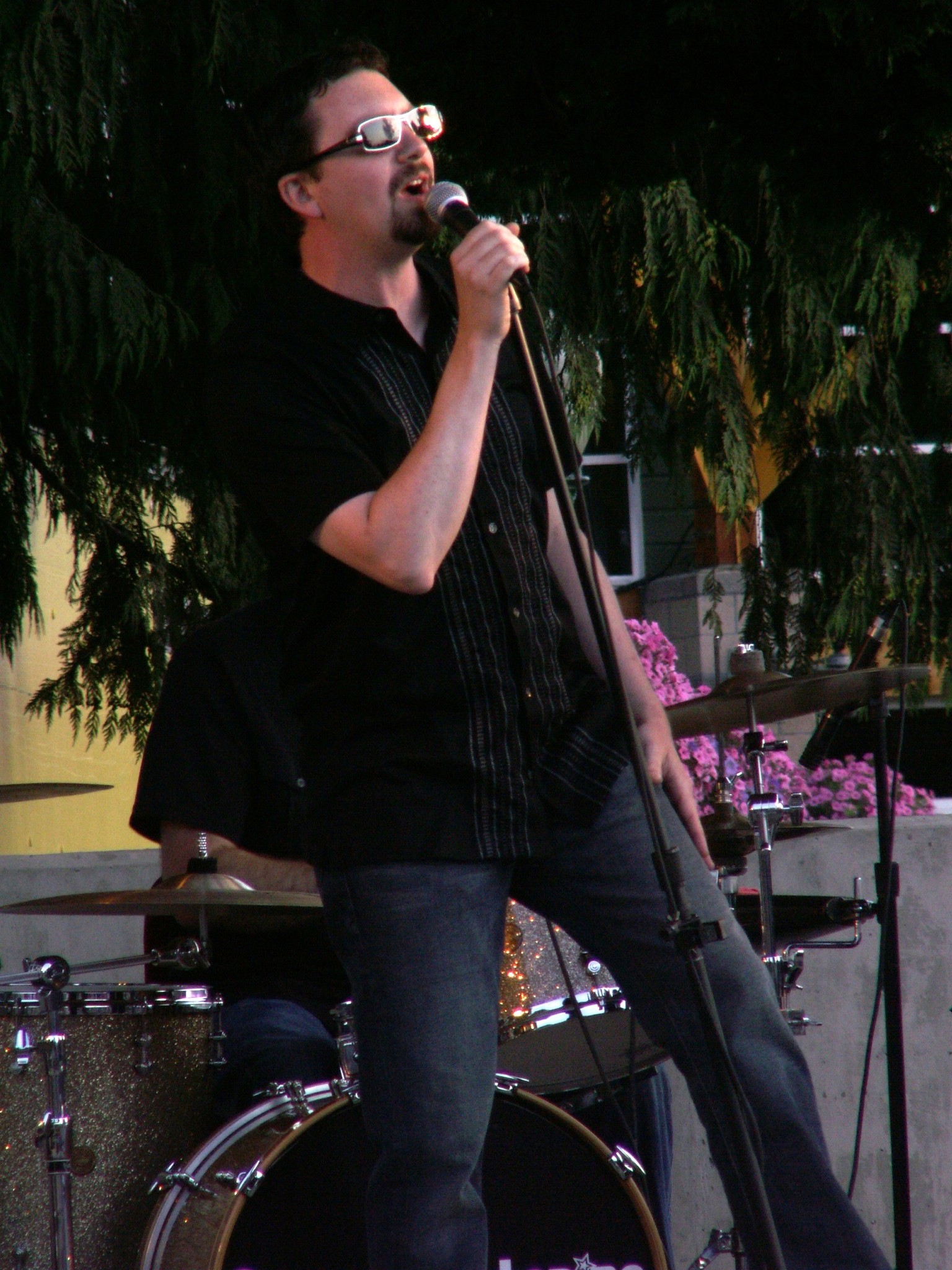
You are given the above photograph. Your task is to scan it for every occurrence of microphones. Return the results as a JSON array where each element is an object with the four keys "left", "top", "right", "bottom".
[{"left": 424, "top": 181, "right": 534, "bottom": 292}]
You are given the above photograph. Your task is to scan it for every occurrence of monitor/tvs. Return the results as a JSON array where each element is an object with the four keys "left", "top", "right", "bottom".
[{"left": 572, "top": 452, "right": 646, "bottom": 586}]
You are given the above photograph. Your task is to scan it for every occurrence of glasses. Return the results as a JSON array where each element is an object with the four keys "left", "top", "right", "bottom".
[{"left": 296, "top": 103, "right": 446, "bottom": 172}]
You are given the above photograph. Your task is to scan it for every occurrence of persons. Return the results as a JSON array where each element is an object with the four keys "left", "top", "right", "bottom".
[
  {"left": 127, "top": 593, "right": 354, "bottom": 1004},
  {"left": 254, "top": 27, "right": 891, "bottom": 1270}
]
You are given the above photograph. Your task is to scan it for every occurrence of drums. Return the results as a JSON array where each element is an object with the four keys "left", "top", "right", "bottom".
[
  {"left": 0, "top": 975, "right": 225, "bottom": 1270},
  {"left": 495, "top": 895, "right": 680, "bottom": 1095},
  {"left": 138, "top": 1072, "right": 668, "bottom": 1270}
]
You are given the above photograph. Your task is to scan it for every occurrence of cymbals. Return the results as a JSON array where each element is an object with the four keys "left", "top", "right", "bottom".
[
  {"left": 0, "top": 781, "right": 114, "bottom": 804},
  {"left": 0, "top": 872, "right": 330, "bottom": 923},
  {"left": 687, "top": 802, "right": 855, "bottom": 872},
  {"left": 661, "top": 647, "right": 936, "bottom": 736}
]
